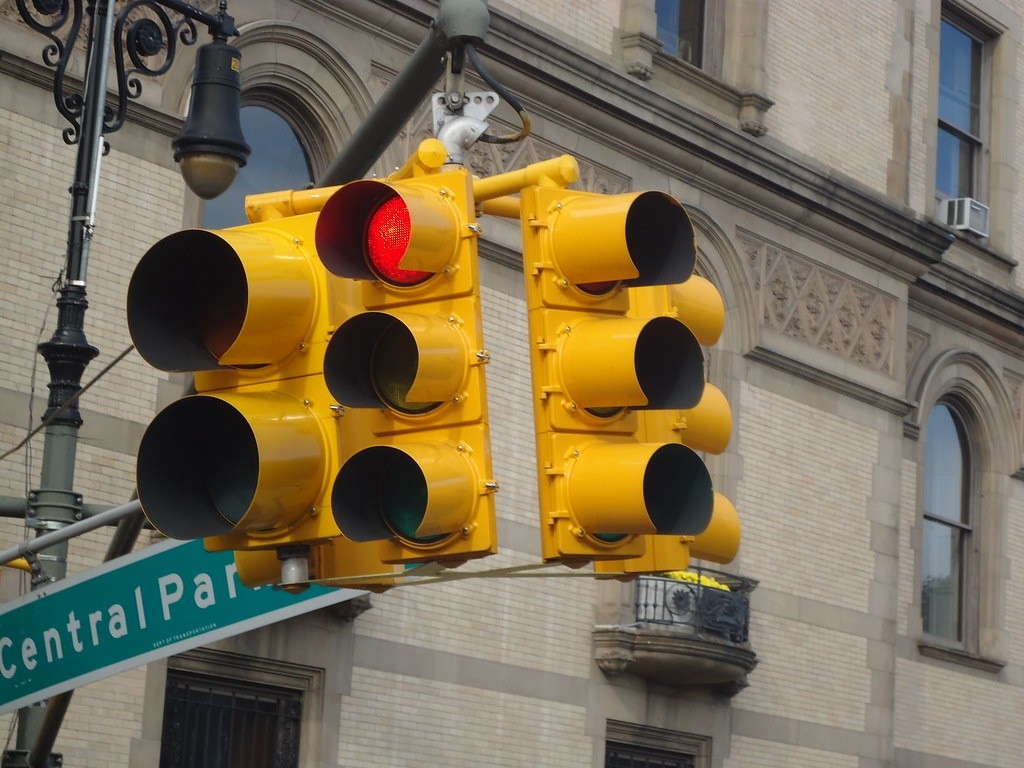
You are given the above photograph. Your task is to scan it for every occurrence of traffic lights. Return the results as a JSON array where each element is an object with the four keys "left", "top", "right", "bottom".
[
  {"left": 520, "top": 185, "right": 741, "bottom": 583},
  {"left": 126, "top": 168, "right": 499, "bottom": 587}
]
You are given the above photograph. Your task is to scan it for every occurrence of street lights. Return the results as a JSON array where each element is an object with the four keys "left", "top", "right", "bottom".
[{"left": 0, "top": 0, "right": 252, "bottom": 767}]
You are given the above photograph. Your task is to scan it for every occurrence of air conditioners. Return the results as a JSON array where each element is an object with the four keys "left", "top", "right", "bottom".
[{"left": 938, "top": 197, "right": 990, "bottom": 238}]
